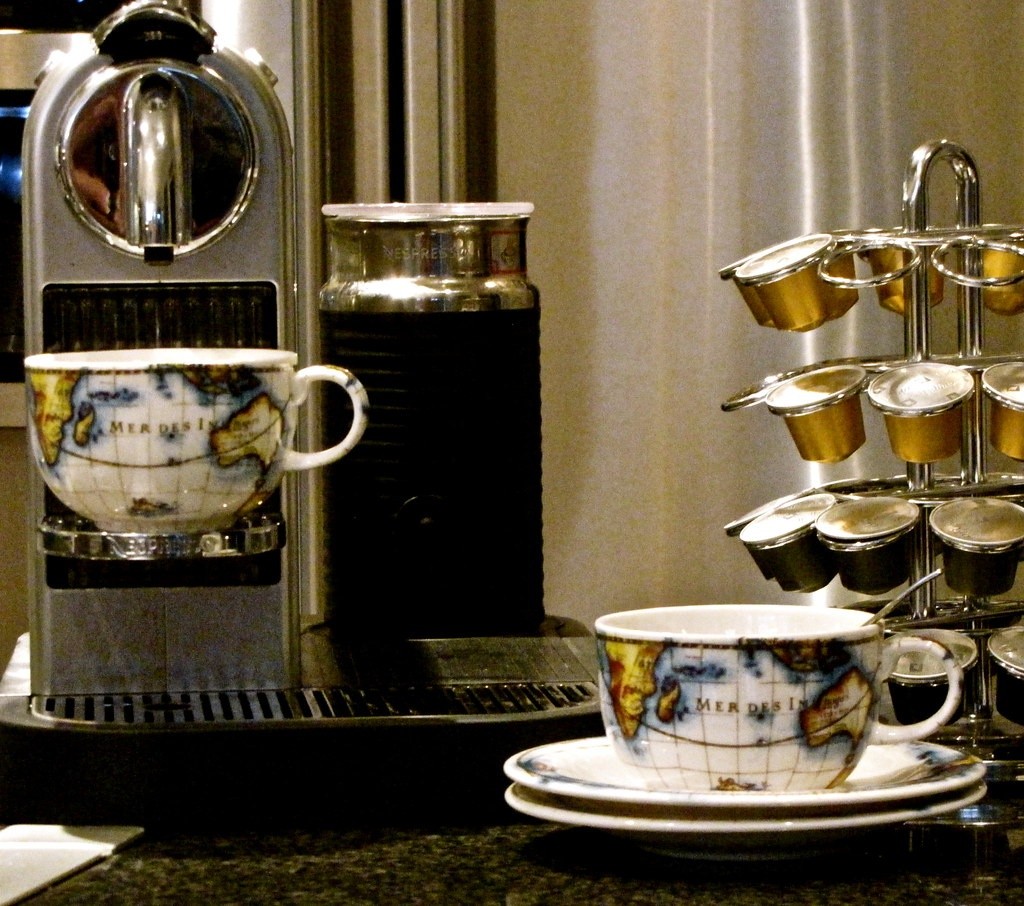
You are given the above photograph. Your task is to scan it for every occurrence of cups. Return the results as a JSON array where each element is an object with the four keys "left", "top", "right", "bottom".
[
  {"left": 594, "top": 604, "right": 963, "bottom": 794},
  {"left": 23, "top": 347, "right": 368, "bottom": 530}
]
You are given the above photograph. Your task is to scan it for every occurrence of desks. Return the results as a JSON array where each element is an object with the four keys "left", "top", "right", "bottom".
[{"left": 0, "top": 624, "right": 1024, "bottom": 906}]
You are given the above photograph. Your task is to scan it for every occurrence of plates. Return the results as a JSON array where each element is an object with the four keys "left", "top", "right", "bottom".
[{"left": 502, "top": 738, "right": 986, "bottom": 860}]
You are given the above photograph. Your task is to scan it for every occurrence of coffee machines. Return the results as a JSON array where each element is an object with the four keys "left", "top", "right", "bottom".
[{"left": 0, "top": 0, "right": 596, "bottom": 771}]
